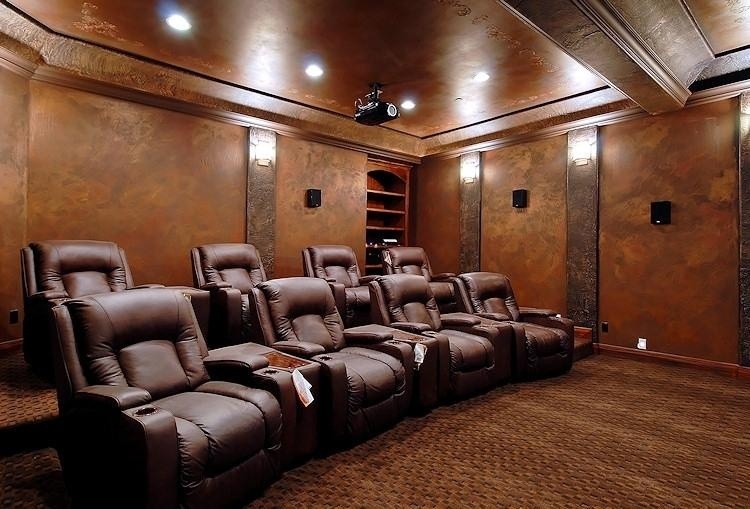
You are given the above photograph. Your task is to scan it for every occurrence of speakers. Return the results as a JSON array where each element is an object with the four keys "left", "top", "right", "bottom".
[
  {"left": 651, "top": 201, "right": 671, "bottom": 224},
  {"left": 513, "top": 190, "right": 527, "bottom": 208},
  {"left": 307, "top": 189, "right": 321, "bottom": 208}
]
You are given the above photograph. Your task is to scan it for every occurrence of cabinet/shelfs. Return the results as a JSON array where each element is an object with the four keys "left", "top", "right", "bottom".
[{"left": 366, "top": 157, "right": 413, "bottom": 275}]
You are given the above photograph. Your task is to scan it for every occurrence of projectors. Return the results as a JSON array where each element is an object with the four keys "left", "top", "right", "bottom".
[{"left": 354, "top": 102, "right": 398, "bottom": 126}]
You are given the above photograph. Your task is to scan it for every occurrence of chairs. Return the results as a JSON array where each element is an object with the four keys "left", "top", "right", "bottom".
[
  {"left": 190, "top": 242, "right": 268, "bottom": 332},
  {"left": 252, "top": 275, "right": 416, "bottom": 447},
  {"left": 368, "top": 274, "right": 506, "bottom": 406},
  {"left": 18, "top": 239, "right": 207, "bottom": 362},
  {"left": 449, "top": 270, "right": 575, "bottom": 382},
  {"left": 382, "top": 246, "right": 457, "bottom": 313},
  {"left": 303, "top": 244, "right": 380, "bottom": 324},
  {"left": 48, "top": 286, "right": 305, "bottom": 506}
]
